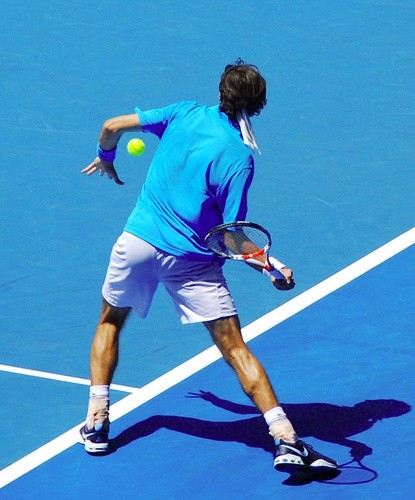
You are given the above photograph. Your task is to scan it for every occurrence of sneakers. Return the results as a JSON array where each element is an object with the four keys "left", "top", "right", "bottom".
[
  {"left": 76, "top": 422, "right": 110, "bottom": 453},
  {"left": 273, "top": 439, "right": 338, "bottom": 474}
]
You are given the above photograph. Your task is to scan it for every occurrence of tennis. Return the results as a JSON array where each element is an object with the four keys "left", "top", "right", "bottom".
[{"left": 127, "top": 139, "right": 144, "bottom": 155}]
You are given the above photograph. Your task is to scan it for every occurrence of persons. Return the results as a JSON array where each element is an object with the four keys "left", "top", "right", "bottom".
[{"left": 78, "top": 59, "right": 338, "bottom": 475}]
